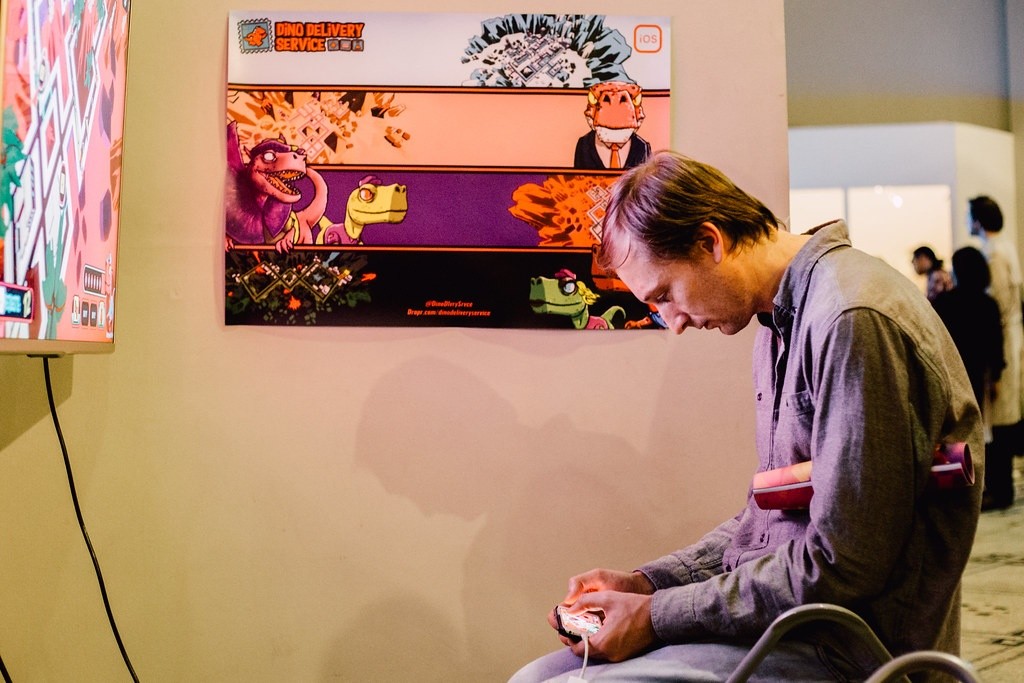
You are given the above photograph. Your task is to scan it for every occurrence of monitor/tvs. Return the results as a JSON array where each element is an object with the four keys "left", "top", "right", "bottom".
[{"left": 0, "top": 0, "right": 132, "bottom": 359}]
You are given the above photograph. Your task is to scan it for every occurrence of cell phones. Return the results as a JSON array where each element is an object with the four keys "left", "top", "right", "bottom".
[{"left": 554, "top": 604, "right": 602, "bottom": 641}]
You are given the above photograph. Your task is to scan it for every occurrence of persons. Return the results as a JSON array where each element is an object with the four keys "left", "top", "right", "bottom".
[
  {"left": 503, "top": 150, "right": 985, "bottom": 683},
  {"left": 911, "top": 196, "right": 1024, "bottom": 513}
]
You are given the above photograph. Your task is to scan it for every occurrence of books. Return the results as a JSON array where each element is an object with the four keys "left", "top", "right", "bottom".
[{"left": 753, "top": 442, "right": 974, "bottom": 510}]
